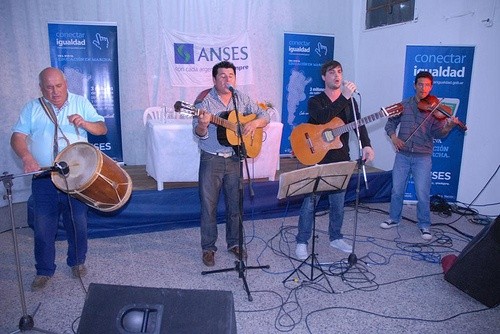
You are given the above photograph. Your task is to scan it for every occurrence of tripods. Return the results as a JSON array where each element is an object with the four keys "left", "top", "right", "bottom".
[
  {"left": 0, "top": 166, "right": 62, "bottom": 334},
  {"left": 277, "top": 95, "right": 372, "bottom": 293},
  {"left": 201, "top": 94, "right": 269, "bottom": 301}
]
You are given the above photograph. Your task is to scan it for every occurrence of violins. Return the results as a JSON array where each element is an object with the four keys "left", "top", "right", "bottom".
[{"left": 418, "top": 92, "right": 468, "bottom": 131}]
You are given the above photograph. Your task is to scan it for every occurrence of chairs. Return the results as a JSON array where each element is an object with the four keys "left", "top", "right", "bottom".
[
  {"left": 265, "top": 106, "right": 280, "bottom": 171},
  {"left": 143, "top": 107, "right": 180, "bottom": 128}
]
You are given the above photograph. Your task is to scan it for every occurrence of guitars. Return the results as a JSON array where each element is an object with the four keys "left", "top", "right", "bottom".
[
  {"left": 289, "top": 103, "right": 405, "bottom": 167},
  {"left": 173, "top": 101, "right": 263, "bottom": 157}
]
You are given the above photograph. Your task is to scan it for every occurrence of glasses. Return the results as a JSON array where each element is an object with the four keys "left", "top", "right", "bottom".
[{"left": 417, "top": 84, "right": 432, "bottom": 88}]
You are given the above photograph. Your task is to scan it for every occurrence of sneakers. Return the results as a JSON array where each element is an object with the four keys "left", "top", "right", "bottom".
[
  {"left": 419, "top": 228, "right": 432, "bottom": 239},
  {"left": 381, "top": 219, "right": 400, "bottom": 228}
]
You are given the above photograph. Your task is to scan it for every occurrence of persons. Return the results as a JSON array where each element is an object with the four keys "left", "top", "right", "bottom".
[
  {"left": 381, "top": 71, "right": 459, "bottom": 239},
  {"left": 295, "top": 60, "right": 373, "bottom": 260},
  {"left": 11, "top": 67, "right": 108, "bottom": 291},
  {"left": 192, "top": 61, "right": 270, "bottom": 268}
]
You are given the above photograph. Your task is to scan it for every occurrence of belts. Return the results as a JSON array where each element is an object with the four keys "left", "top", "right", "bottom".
[{"left": 202, "top": 149, "right": 235, "bottom": 158}]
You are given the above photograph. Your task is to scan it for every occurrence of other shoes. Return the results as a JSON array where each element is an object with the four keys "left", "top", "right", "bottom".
[
  {"left": 31, "top": 274, "right": 49, "bottom": 290},
  {"left": 232, "top": 246, "right": 247, "bottom": 259},
  {"left": 203, "top": 250, "right": 214, "bottom": 266},
  {"left": 330, "top": 239, "right": 352, "bottom": 253},
  {"left": 71, "top": 263, "right": 86, "bottom": 277},
  {"left": 296, "top": 244, "right": 308, "bottom": 259}
]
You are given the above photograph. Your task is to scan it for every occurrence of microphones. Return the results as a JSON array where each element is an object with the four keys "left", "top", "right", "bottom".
[
  {"left": 54, "top": 162, "right": 58, "bottom": 171},
  {"left": 226, "top": 83, "right": 237, "bottom": 95},
  {"left": 343, "top": 80, "right": 360, "bottom": 95}
]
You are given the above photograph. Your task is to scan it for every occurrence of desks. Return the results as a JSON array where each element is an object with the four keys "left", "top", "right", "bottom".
[{"left": 145, "top": 120, "right": 283, "bottom": 194}]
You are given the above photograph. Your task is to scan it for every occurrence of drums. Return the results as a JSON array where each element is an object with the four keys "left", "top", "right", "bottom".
[{"left": 50, "top": 140, "right": 133, "bottom": 213}]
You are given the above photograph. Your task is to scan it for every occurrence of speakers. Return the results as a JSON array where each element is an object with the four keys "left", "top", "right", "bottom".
[
  {"left": 444, "top": 219, "right": 500, "bottom": 309},
  {"left": 77, "top": 283, "right": 238, "bottom": 334}
]
